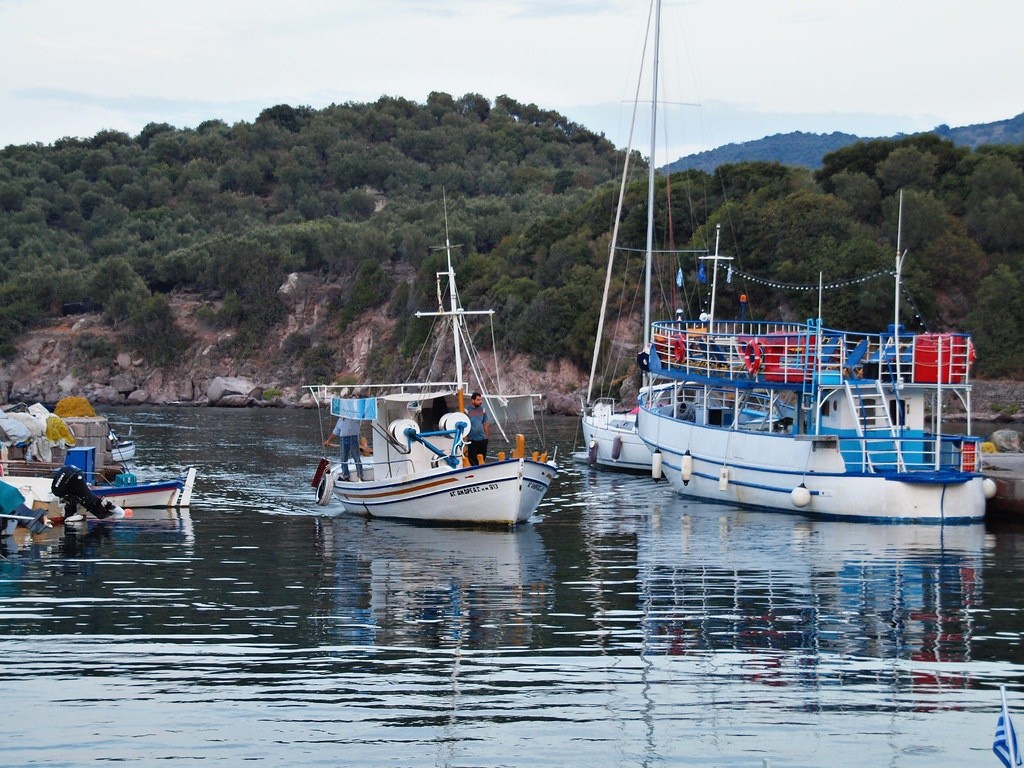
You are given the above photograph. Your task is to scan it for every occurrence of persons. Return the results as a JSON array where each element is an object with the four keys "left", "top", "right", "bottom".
[
  {"left": 466, "top": 392, "right": 490, "bottom": 468},
  {"left": 323, "top": 416, "right": 365, "bottom": 482}
]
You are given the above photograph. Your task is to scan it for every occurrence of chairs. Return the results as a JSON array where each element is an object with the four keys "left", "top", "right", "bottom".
[
  {"left": 821, "top": 337, "right": 912, "bottom": 380},
  {"left": 697, "top": 339, "right": 742, "bottom": 378}
]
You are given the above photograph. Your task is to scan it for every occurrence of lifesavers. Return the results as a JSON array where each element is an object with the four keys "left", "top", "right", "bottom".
[
  {"left": 744, "top": 339, "right": 760, "bottom": 375},
  {"left": 674, "top": 339, "right": 686, "bottom": 362},
  {"left": 315, "top": 473, "right": 334, "bottom": 506},
  {"left": 962, "top": 340, "right": 974, "bottom": 372}
]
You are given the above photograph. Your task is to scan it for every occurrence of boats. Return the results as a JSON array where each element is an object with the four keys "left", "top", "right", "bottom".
[
  {"left": 302, "top": 239, "right": 560, "bottom": 526},
  {"left": 579, "top": 0, "right": 996, "bottom": 524},
  {"left": 108, "top": 439, "right": 138, "bottom": 461},
  {"left": 0, "top": 463, "right": 197, "bottom": 509}
]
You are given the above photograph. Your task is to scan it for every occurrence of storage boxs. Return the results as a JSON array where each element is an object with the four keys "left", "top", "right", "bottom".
[{"left": 115, "top": 474, "right": 137, "bottom": 487}]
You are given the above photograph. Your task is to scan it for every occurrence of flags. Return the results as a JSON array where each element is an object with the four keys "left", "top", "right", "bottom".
[
  {"left": 675, "top": 266, "right": 684, "bottom": 288},
  {"left": 696, "top": 259, "right": 707, "bottom": 284}
]
